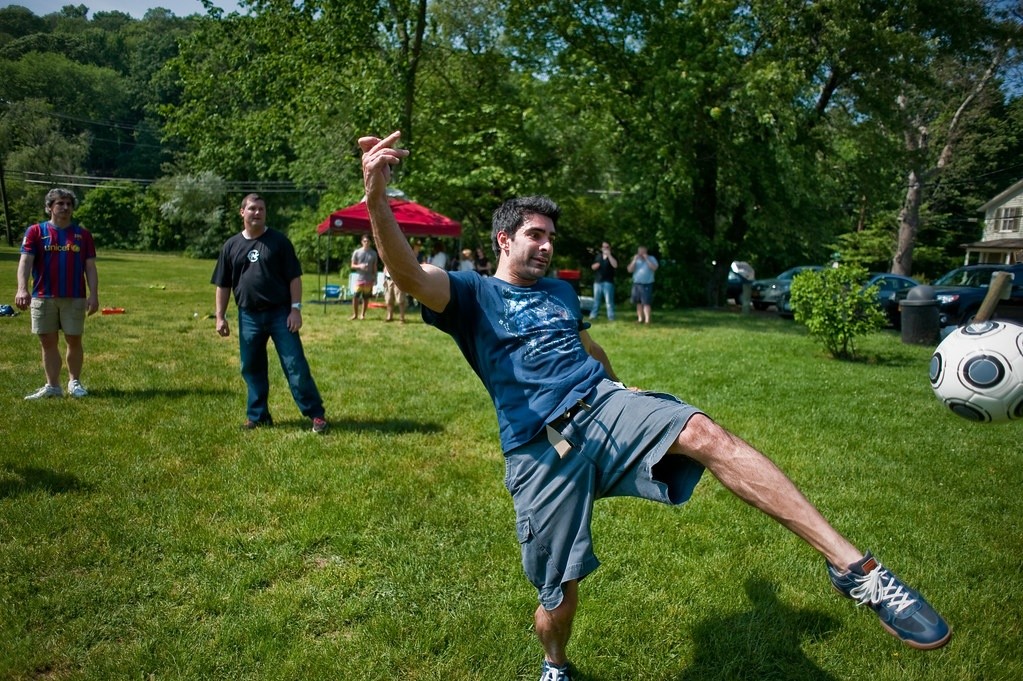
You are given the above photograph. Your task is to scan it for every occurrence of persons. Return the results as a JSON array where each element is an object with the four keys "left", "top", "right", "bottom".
[
  {"left": 356, "top": 131, "right": 950, "bottom": 681},
  {"left": 14, "top": 188, "right": 100, "bottom": 402},
  {"left": 350, "top": 234, "right": 378, "bottom": 321},
  {"left": 589, "top": 238, "right": 618, "bottom": 321},
  {"left": 451, "top": 243, "right": 493, "bottom": 277},
  {"left": 413, "top": 241, "right": 447, "bottom": 270},
  {"left": 384, "top": 264, "right": 406, "bottom": 322},
  {"left": 209, "top": 194, "right": 328, "bottom": 435},
  {"left": 625, "top": 246, "right": 659, "bottom": 325}
]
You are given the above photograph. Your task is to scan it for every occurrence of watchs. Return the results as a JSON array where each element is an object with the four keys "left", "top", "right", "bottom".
[{"left": 291, "top": 303, "right": 302, "bottom": 310}]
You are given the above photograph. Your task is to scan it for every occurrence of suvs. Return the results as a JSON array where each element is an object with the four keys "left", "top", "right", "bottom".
[{"left": 894, "top": 263, "right": 1023, "bottom": 331}]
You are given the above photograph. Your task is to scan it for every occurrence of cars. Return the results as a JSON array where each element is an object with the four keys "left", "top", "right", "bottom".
[
  {"left": 753, "top": 265, "right": 828, "bottom": 310},
  {"left": 778, "top": 272, "right": 920, "bottom": 327}
]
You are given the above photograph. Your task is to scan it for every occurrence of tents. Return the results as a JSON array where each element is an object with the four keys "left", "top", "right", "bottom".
[{"left": 317, "top": 194, "right": 462, "bottom": 313}]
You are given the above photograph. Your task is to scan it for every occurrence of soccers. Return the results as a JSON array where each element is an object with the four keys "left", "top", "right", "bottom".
[{"left": 929, "top": 320, "right": 1023, "bottom": 425}]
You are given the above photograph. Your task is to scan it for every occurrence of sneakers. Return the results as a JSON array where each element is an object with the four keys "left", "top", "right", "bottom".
[
  {"left": 68, "top": 379, "right": 88, "bottom": 396},
  {"left": 539, "top": 661, "right": 571, "bottom": 681},
  {"left": 24, "top": 384, "right": 63, "bottom": 400},
  {"left": 312, "top": 416, "right": 326, "bottom": 433},
  {"left": 243, "top": 419, "right": 273, "bottom": 428},
  {"left": 827, "top": 548, "right": 951, "bottom": 649}
]
